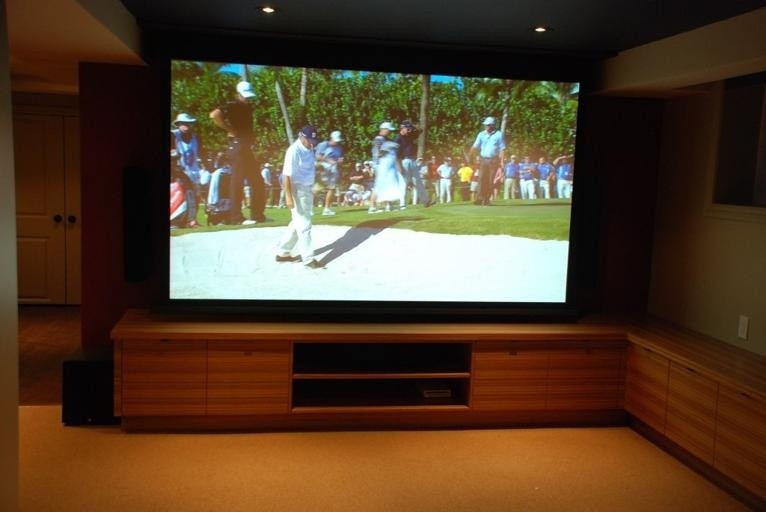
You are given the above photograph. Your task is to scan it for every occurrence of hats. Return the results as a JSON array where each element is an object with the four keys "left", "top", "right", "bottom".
[
  {"left": 378, "top": 120, "right": 397, "bottom": 133},
  {"left": 481, "top": 116, "right": 496, "bottom": 127},
  {"left": 417, "top": 155, "right": 570, "bottom": 162},
  {"left": 400, "top": 120, "right": 414, "bottom": 130},
  {"left": 329, "top": 129, "right": 343, "bottom": 144},
  {"left": 355, "top": 160, "right": 374, "bottom": 167},
  {"left": 299, "top": 124, "right": 319, "bottom": 145},
  {"left": 262, "top": 161, "right": 274, "bottom": 168},
  {"left": 173, "top": 112, "right": 197, "bottom": 126},
  {"left": 235, "top": 80, "right": 257, "bottom": 98}
]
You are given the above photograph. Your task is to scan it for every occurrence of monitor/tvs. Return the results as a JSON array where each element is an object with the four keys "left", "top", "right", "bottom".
[{"left": 167, "top": 58, "right": 581, "bottom": 316}]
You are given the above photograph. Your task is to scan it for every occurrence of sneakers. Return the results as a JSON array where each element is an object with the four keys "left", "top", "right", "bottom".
[
  {"left": 274, "top": 252, "right": 301, "bottom": 264},
  {"left": 425, "top": 198, "right": 438, "bottom": 209},
  {"left": 188, "top": 221, "right": 203, "bottom": 228},
  {"left": 472, "top": 199, "right": 491, "bottom": 206},
  {"left": 367, "top": 205, "right": 408, "bottom": 214},
  {"left": 322, "top": 208, "right": 335, "bottom": 216},
  {"left": 304, "top": 257, "right": 325, "bottom": 269},
  {"left": 240, "top": 216, "right": 275, "bottom": 226}
]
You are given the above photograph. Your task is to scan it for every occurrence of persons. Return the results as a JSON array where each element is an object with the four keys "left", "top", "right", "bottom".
[{"left": 172, "top": 81, "right": 573, "bottom": 269}]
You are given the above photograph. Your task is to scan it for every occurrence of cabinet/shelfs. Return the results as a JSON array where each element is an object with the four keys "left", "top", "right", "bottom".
[{"left": 106, "top": 305, "right": 765, "bottom": 512}]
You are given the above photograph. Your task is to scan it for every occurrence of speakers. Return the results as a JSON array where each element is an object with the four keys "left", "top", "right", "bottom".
[
  {"left": 122, "top": 167, "right": 152, "bottom": 281},
  {"left": 62, "top": 359, "right": 122, "bottom": 427}
]
